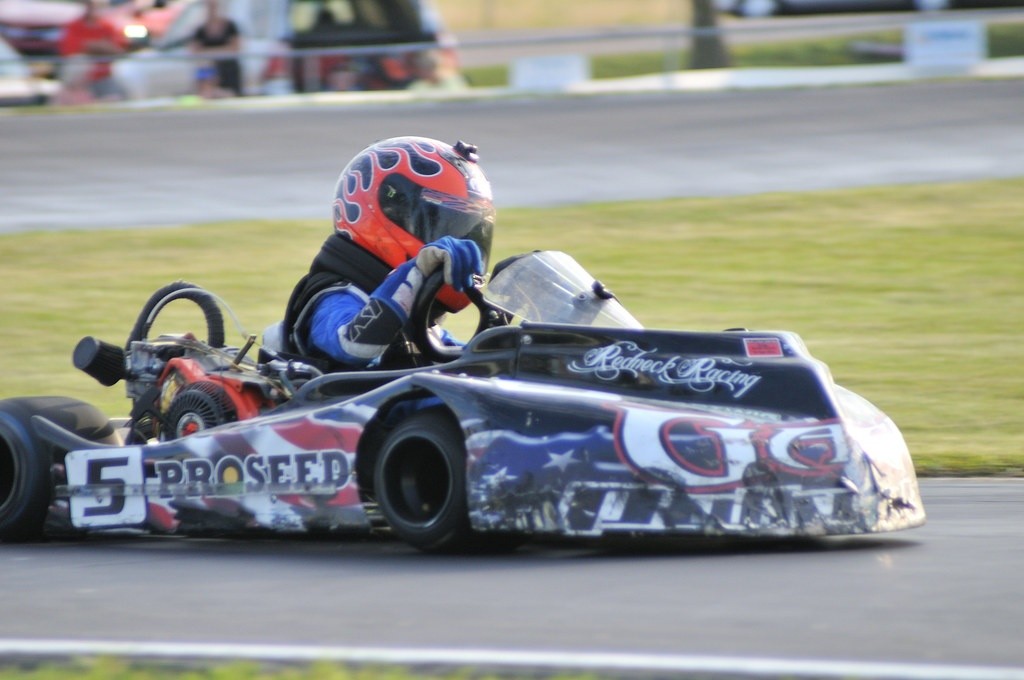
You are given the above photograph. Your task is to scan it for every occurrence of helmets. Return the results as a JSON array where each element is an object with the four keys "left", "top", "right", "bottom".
[{"left": 331, "top": 135, "right": 495, "bottom": 292}]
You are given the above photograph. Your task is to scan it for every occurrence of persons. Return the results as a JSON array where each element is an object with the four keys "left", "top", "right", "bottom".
[
  {"left": 282, "top": 136, "right": 495, "bottom": 394},
  {"left": 58, "top": 0, "right": 129, "bottom": 99},
  {"left": 195, "top": 0, "right": 243, "bottom": 96}
]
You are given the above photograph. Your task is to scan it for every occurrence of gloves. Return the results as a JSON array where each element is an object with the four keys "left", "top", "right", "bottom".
[{"left": 368, "top": 235, "right": 484, "bottom": 327}]
